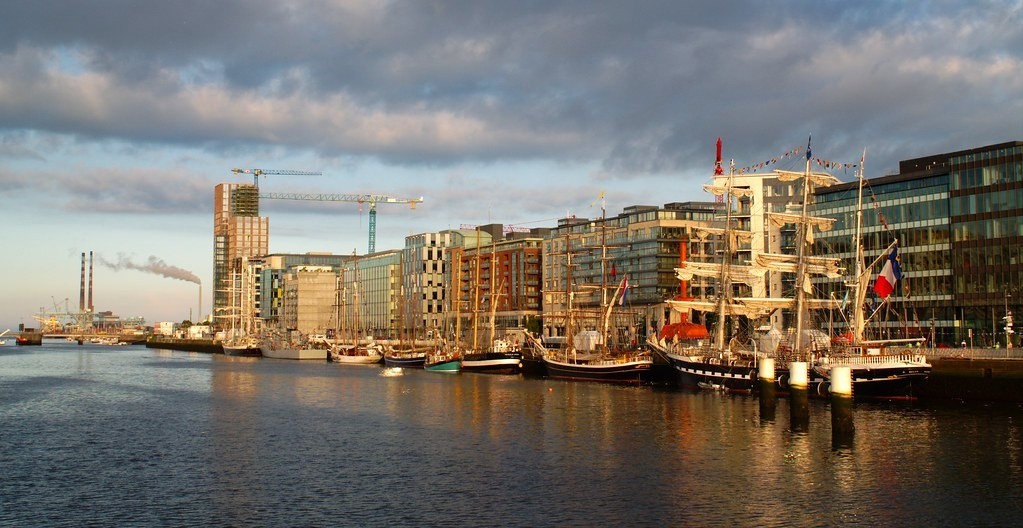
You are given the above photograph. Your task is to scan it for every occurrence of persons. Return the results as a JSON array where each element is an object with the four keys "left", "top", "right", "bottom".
[
  {"left": 961, "top": 339, "right": 967, "bottom": 347},
  {"left": 954, "top": 341, "right": 958, "bottom": 347},
  {"left": 995, "top": 342, "right": 999, "bottom": 349},
  {"left": 916, "top": 341, "right": 921, "bottom": 348},
  {"left": 516, "top": 337, "right": 519, "bottom": 344},
  {"left": 907, "top": 342, "right": 912, "bottom": 348}
]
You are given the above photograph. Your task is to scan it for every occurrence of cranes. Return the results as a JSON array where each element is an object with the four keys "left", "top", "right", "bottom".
[
  {"left": 257, "top": 191, "right": 425, "bottom": 253},
  {"left": 229, "top": 167, "right": 324, "bottom": 187}
]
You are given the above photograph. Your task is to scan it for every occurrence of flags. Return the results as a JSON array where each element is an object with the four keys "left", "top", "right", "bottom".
[
  {"left": 861, "top": 153, "right": 867, "bottom": 164},
  {"left": 806, "top": 134, "right": 813, "bottom": 161},
  {"left": 842, "top": 289, "right": 849, "bottom": 310},
  {"left": 873, "top": 245, "right": 905, "bottom": 300},
  {"left": 869, "top": 289, "right": 876, "bottom": 315},
  {"left": 618, "top": 278, "right": 629, "bottom": 307},
  {"left": 902, "top": 272, "right": 910, "bottom": 299},
  {"left": 610, "top": 263, "right": 616, "bottom": 281}
]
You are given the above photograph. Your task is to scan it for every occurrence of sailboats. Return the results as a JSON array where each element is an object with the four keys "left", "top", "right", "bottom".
[
  {"left": 219, "top": 267, "right": 262, "bottom": 357},
  {"left": 640, "top": 127, "right": 933, "bottom": 403},
  {"left": 329, "top": 248, "right": 383, "bottom": 366},
  {"left": 260, "top": 329, "right": 330, "bottom": 360},
  {"left": 459, "top": 227, "right": 546, "bottom": 378},
  {"left": 382, "top": 246, "right": 429, "bottom": 369},
  {"left": 539, "top": 192, "right": 678, "bottom": 387},
  {"left": 424, "top": 296, "right": 463, "bottom": 371}
]
